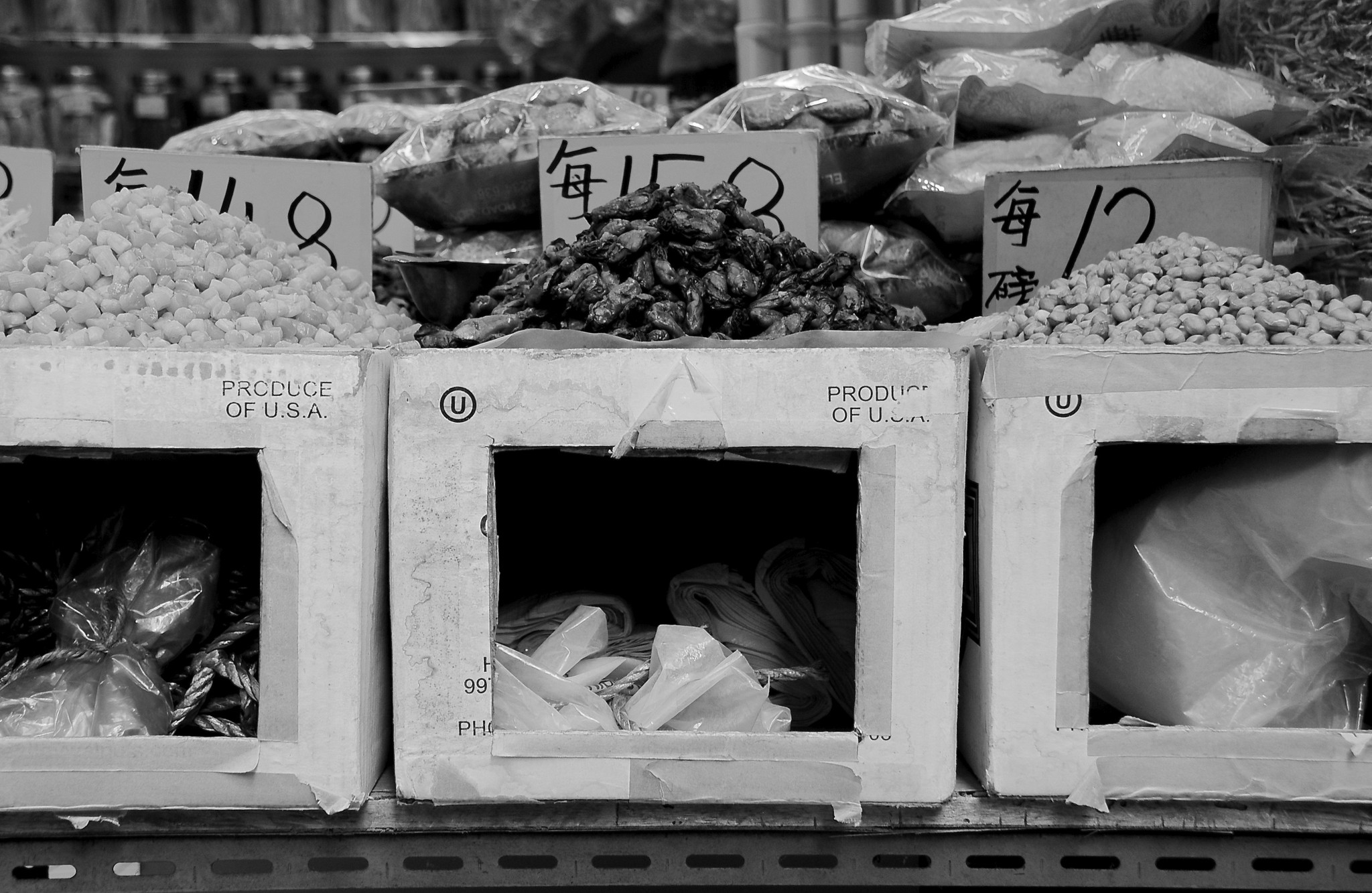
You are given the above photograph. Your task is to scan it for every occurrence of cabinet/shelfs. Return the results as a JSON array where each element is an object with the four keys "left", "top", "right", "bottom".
[
  {"left": 0, "top": 797, "right": 1372, "bottom": 893},
  {"left": 2, "top": 0, "right": 899, "bottom": 224}
]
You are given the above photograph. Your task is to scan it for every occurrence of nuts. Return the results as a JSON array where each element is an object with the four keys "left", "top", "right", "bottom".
[{"left": 948, "top": 232, "right": 1372, "bottom": 350}]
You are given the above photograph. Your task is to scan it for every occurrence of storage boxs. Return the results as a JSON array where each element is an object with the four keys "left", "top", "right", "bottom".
[
  {"left": 2, "top": 338, "right": 402, "bottom": 820},
  {"left": 920, "top": 295, "right": 1372, "bottom": 825},
  {"left": 388, "top": 302, "right": 973, "bottom": 827}
]
